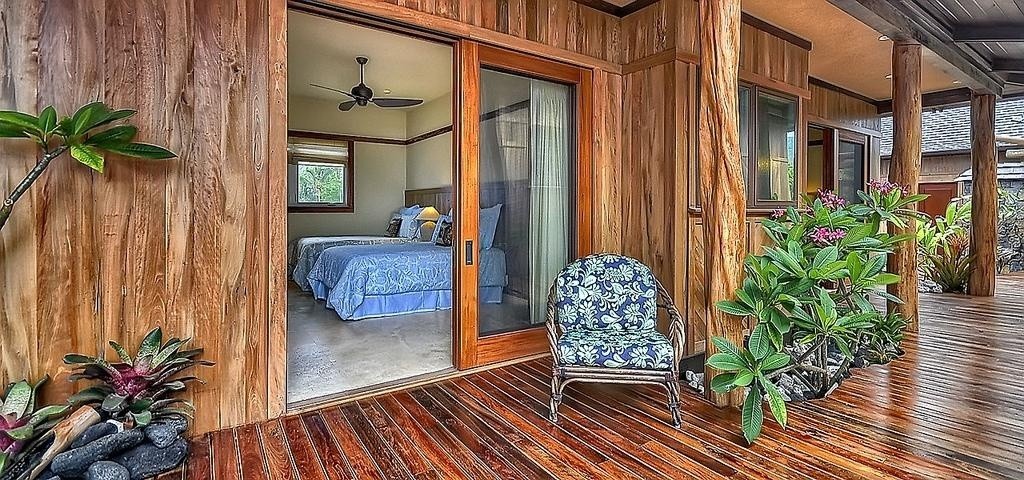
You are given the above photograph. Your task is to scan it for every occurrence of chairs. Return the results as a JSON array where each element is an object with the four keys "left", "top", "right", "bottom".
[{"left": 546, "top": 253, "right": 689, "bottom": 430}]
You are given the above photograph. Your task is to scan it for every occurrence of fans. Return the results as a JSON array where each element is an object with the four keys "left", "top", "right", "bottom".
[{"left": 311, "top": 56, "right": 426, "bottom": 113}]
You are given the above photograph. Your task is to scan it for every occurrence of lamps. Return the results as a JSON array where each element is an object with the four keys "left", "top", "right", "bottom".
[{"left": 413, "top": 206, "right": 440, "bottom": 242}]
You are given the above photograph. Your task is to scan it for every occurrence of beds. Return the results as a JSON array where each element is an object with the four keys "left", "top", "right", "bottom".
[
  {"left": 287, "top": 235, "right": 423, "bottom": 291},
  {"left": 305, "top": 242, "right": 507, "bottom": 320}
]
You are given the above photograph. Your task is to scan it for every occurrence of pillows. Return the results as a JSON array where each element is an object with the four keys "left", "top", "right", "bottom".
[
  {"left": 431, "top": 202, "right": 503, "bottom": 251},
  {"left": 384, "top": 204, "right": 424, "bottom": 238}
]
